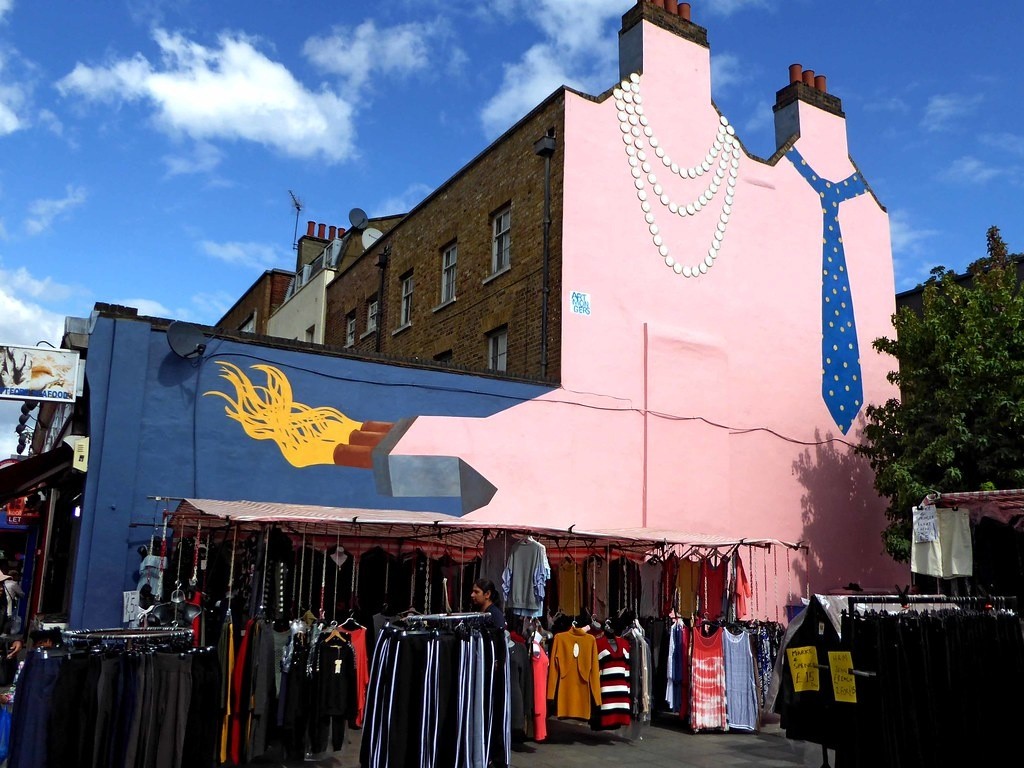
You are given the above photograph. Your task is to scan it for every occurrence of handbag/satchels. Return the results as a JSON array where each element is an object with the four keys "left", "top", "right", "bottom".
[{"left": 12, "top": 599, "right": 18, "bottom": 612}]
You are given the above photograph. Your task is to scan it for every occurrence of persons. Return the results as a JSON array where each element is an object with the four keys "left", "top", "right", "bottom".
[
  {"left": 132, "top": 535, "right": 355, "bottom": 639},
  {"left": 471, "top": 577, "right": 505, "bottom": 633},
  {"left": 0, "top": 626, "right": 63, "bottom": 709},
  {"left": 0, "top": 555, "right": 26, "bottom": 705}
]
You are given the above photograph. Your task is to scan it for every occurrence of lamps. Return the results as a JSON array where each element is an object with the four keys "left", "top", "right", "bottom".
[{"left": 16, "top": 400, "right": 42, "bottom": 453}]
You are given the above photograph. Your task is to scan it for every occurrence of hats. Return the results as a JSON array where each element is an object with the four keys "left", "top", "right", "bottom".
[{"left": 0, "top": 570, "right": 13, "bottom": 581}]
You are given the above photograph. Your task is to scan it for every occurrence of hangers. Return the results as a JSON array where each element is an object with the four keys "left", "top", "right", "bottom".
[
  {"left": 36, "top": 532, "right": 781, "bottom": 659},
  {"left": 840, "top": 595, "right": 1016, "bottom": 623}
]
